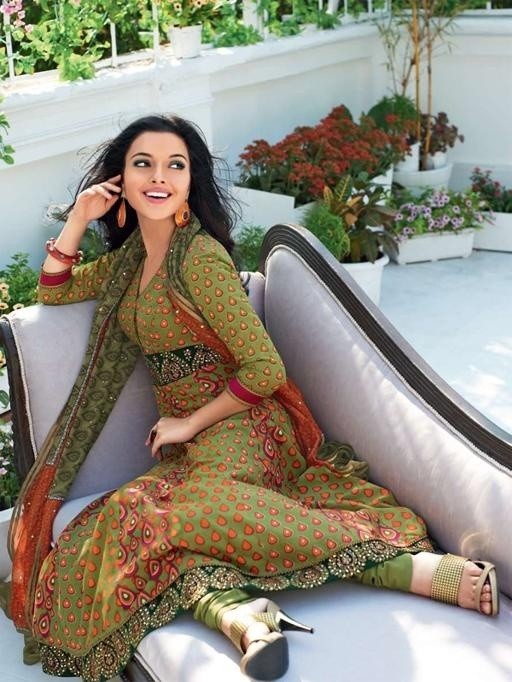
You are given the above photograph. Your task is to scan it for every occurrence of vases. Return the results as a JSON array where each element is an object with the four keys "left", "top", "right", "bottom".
[
  {"left": 472, "top": 212, "right": 512, "bottom": 252},
  {"left": 382, "top": 224, "right": 475, "bottom": 267},
  {"left": 170, "top": 24, "right": 203, "bottom": 59},
  {"left": 0, "top": 504, "right": 17, "bottom": 585}
]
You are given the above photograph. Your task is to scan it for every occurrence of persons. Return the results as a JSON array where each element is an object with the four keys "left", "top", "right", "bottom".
[{"left": 2, "top": 115, "right": 499, "bottom": 679}]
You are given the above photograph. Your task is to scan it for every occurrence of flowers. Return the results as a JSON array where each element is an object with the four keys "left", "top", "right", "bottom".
[
  {"left": 0, "top": 248, "right": 47, "bottom": 376},
  {"left": 155, "top": 0, "right": 226, "bottom": 27},
  {"left": 239, "top": 103, "right": 512, "bottom": 239},
  {"left": 0, "top": 422, "right": 31, "bottom": 517}
]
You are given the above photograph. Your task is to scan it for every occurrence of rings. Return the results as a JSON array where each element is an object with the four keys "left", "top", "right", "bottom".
[{"left": 150, "top": 430, "right": 156, "bottom": 441}]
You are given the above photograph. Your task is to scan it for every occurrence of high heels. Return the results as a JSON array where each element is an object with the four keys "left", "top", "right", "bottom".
[
  {"left": 228, "top": 598, "right": 313, "bottom": 680},
  {"left": 430, "top": 551, "right": 498, "bottom": 617}
]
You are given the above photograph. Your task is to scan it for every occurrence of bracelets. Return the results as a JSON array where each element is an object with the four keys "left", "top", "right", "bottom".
[{"left": 44, "top": 237, "right": 84, "bottom": 266}]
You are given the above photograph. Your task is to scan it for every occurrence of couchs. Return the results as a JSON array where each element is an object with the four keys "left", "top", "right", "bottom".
[{"left": 0, "top": 212, "right": 512, "bottom": 681}]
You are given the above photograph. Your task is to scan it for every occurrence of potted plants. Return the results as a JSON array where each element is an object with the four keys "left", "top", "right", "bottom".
[
  {"left": 370, "top": 1, "right": 477, "bottom": 197},
  {"left": 323, "top": 174, "right": 401, "bottom": 306}
]
